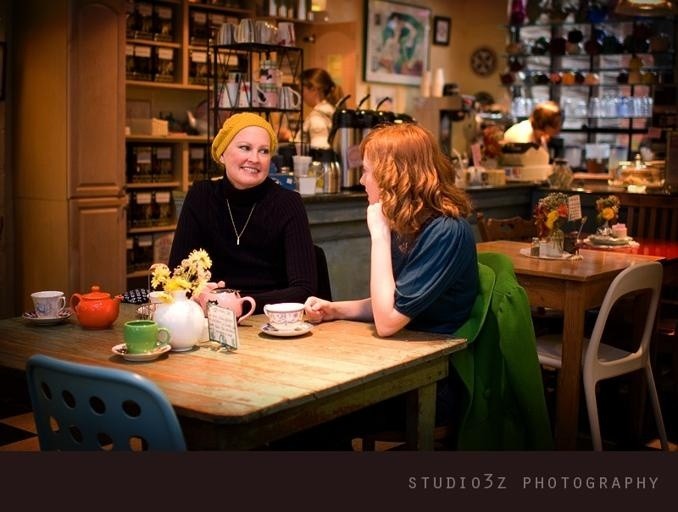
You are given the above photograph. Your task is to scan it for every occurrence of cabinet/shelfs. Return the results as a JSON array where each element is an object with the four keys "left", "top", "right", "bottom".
[
  {"left": 509, "top": 17, "right": 678, "bottom": 135},
  {"left": 14, "top": 1, "right": 298, "bottom": 319},
  {"left": 207, "top": 43, "right": 303, "bottom": 179}
]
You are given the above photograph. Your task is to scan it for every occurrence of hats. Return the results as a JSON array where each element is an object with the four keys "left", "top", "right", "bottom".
[{"left": 212, "top": 112, "right": 279, "bottom": 166}]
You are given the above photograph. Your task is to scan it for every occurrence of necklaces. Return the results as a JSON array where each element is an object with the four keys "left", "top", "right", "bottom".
[{"left": 227, "top": 199, "right": 256, "bottom": 246}]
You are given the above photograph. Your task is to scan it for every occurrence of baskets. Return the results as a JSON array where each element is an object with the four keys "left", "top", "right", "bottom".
[{"left": 121, "top": 288, "right": 153, "bottom": 304}]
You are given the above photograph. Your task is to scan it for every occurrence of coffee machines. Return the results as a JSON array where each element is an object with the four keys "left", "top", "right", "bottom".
[{"left": 411, "top": 96, "right": 478, "bottom": 189}]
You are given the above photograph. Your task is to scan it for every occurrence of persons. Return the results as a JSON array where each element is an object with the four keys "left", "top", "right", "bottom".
[
  {"left": 275, "top": 67, "right": 346, "bottom": 149},
  {"left": 441, "top": 84, "right": 565, "bottom": 150},
  {"left": 168, "top": 112, "right": 317, "bottom": 316},
  {"left": 305, "top": 121, "right": 481, "bottom": 450}
]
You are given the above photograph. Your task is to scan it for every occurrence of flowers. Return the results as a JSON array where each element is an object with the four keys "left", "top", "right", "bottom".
[
  {"left": 150, "top": 247, "right": 213, "bottom": 305},
  {"left": 530, "top": 193, "right": 570, "bottom": 254},
  {"left": 595, "top": 194, "right": 624, "bottom": 225}
]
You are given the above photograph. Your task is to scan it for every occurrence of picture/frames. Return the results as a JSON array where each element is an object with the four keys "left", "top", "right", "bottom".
[
  {"left": 362, "top": 0, "right": 432, "bottom": 87},
  {"left": 433, "top": 16, "right": 451, "bottom": 46}
]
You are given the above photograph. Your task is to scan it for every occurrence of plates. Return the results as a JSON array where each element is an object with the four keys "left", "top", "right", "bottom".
[
  {"left": 136, "top": 304, "right": 156, "bottom": 316},
  {"left": 113, "top": 342, "right": 171, "bottom": 361},
  {"left": 589, "top": 235, "right": 631, "bottom": 246},
  {"left": 20, "top": 309, "right": 72, "bottom": 327},
  {"left": 261, "top": 321, "right": 314, "bottom": 338}
]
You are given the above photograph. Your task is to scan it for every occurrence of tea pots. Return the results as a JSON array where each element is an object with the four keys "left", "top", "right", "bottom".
[
  {"left": 69, "top": 284, "right": 126, "bottom": 330},
  {"left": 197, "top": 288, "right": 256, "bottom": 326}
]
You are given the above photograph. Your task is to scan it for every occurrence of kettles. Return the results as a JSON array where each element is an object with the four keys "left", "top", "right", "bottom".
[{"left": 306, "top": 146, "right": 341, "bottom": 193}]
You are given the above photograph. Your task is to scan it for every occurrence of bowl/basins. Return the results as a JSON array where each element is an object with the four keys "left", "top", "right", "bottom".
[{"left": 522, "top": 165, "right": 553, "bottom": 181}]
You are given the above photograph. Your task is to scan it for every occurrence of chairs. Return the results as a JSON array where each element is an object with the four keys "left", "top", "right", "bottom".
[
  {"left": 435, "top": 253, "right": 518, "bottom": 451},
  {"left": 314, "top": 245, "right": 332, "bottom": 301},
  {"left": 475, "top": 193, "right": 678, "bottom": 451},
  {"left": 26, "top": 354, "right": 187, "bottom": 452}
]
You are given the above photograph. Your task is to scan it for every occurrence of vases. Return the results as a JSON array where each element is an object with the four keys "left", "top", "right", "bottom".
[{"left": 152, "top": 290, "right": 206, "bottom": 352}]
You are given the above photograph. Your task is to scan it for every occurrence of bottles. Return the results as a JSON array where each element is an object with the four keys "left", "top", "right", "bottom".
[
  {"left": 530, "top": 237, "right": 540, "bottom": 256},
  {"left": 508, "top": 95, "right": 653, "bottom": 119}
]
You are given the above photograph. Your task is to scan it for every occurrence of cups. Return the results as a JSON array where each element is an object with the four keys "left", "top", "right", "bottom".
[
  {"left": 239, "top": 81, "right": 267, "bottom": 108},
  {"left": 431, "top": 67, "right": 445, "bottom": 98},
  {"left": 481, "top": 169, "right": 505, "bottom": 186},
  {"left": 28, "top": 288, "right": 68, "bottom": 318},
  {"left": 299, "top": 176, "right": 317, "bottom": 196},
  {"left": 261, "top": 302, "right": 307, "bottom": 331},
  {"left": 218, "top": 18, "right": 296, "bottom": 48},
  {"left": 292, "top": 154, "right": 312, "bottom": 177},
  {"left": 259, "top": 59, "right": 283, "bottom": 106},
  {"left": 420, "top": 69, "right": 432, "bottom": 99},
  {"left": 276, "top": 86, "right": 302, "bottom": 110},
  {"left": 121, "top": 320, "right": 170, "bottom": 354},
  {"left": 147, "top": 290, "right": 166, "bottom": 308}
]
